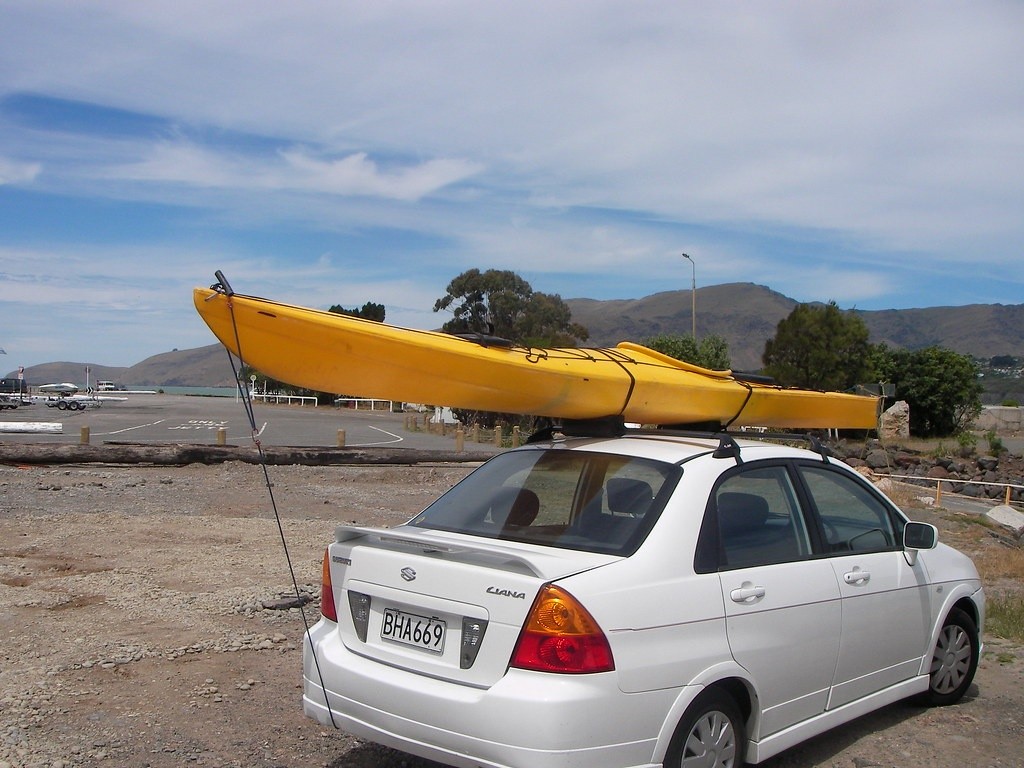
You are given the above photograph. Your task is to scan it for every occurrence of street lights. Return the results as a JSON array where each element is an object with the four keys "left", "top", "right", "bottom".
[{"left": 683, "top": 254, "right": 696, "bottom": 338}]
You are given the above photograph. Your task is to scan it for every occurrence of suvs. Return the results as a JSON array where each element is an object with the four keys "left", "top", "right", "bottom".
[{"left": 302, "top": 430, "right": 988, "bottom": 768}]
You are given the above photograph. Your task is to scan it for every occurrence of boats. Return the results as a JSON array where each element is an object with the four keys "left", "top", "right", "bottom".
[{"left": 193, "top": 286, "right": 883, "bottom": 442}]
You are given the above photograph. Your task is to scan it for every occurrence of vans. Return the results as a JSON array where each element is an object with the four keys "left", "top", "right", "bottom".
[
  {"left": 0, "top": 379, "right": 28, "bottom": 409},
  {"left": 97, "top": 380, "right": 115, "bottom": 390}
]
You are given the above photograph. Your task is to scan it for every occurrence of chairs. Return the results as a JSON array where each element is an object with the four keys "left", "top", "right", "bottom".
[
  {"left": 607, "top": 478, "right": 652, "bottom": 523},
  {"left": 491, "top": 488, "right": 540, "bottom": 528},
  {"left": 718, "top": 493, "right": 769, "bottom": 529}
]
